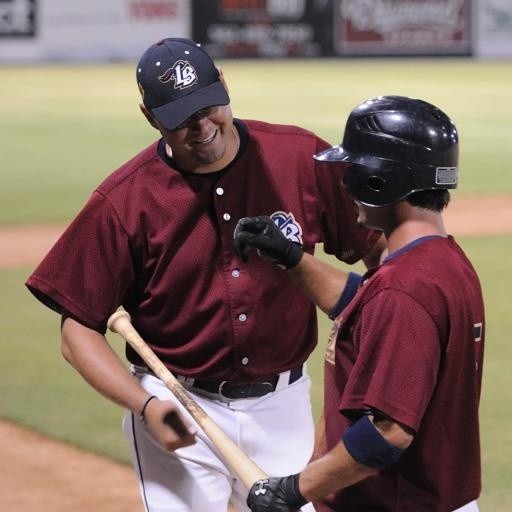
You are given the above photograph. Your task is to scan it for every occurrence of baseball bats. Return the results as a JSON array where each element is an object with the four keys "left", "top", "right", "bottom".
[{"left": 107, "top": 310, "right": 269, "bottom": 489}]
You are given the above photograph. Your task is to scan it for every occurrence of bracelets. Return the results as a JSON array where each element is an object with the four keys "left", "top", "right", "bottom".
[{"left": 140, "top": 395, "right": 157, "bottom": 424}]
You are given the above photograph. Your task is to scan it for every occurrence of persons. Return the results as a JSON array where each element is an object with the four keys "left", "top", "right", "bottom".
[
  {"left": 23, "top": 38, "right": 389, "bottom": 512},
  {"left": 231, "top": 94, "right": 485, "bottom": 512}
]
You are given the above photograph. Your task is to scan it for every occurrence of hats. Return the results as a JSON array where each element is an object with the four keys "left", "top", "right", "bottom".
[{"left": 135, "top": 36, "right": 231, "bottom": 130}]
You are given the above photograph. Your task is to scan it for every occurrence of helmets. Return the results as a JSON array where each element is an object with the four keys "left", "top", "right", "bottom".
[{"left": 311, "top": 93, "right": 463, "bottom": 210}]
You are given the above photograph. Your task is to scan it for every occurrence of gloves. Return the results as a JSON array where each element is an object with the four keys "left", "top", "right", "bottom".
[
  {"left": 246, "top": 472, "right": 309, "bottom": 512},
  {"left": 232, "top": 214, "right": 304, "bottom": 272}
]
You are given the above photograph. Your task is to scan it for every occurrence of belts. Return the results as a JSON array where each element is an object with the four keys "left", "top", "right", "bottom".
[{"left": 175, "top": 364, "right": 311, "bottom": 399}]
